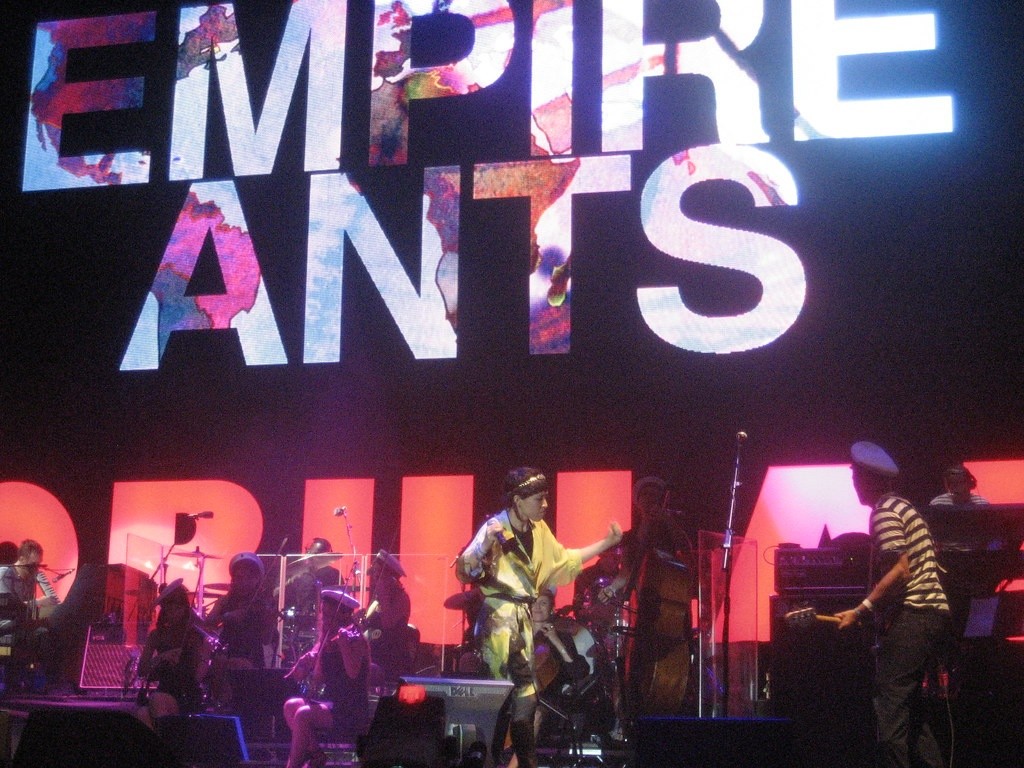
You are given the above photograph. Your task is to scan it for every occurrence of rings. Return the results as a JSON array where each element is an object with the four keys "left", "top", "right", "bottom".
[{"left": 551, "top": 625, "right": 554, "bottom": 629}]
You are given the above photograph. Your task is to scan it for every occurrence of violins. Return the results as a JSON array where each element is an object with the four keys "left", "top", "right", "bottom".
[
  {"left": 237, "top": 594, "right": 287, "bottom": 623},
  {"left": 330, "top": 622, "right": 361, "bottom": 643}
]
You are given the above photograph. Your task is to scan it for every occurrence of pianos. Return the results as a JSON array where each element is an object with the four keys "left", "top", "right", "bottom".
[{"left": 35, "top": 571, "right": 61, "bottom": 606}]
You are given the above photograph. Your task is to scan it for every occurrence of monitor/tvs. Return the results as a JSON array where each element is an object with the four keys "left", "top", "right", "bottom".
[{"left": 398, "top": 677, "right": 518, "bottom": 768}]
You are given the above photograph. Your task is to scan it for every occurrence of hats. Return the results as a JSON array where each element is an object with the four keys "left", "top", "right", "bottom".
[
  {"left": 321, "top": 590, "right": 361, "bottom": 614},
  {"left": 376, "top": 549, "right": 408, "bottom": 579},
  {"left": 153, "top": 578, "right": 187, "bottom": 608},
  {"left": 851, "top": 441, "right": 899, "bottom": 482},
  {"left": 229, "top": 552, "right": 265, "bottom": 579},
  {"left": 542, "top": 586, "right": 557, "bottom": 599},
  {"left": 633, "top": 477, "right": 667, "bottom": 506}
]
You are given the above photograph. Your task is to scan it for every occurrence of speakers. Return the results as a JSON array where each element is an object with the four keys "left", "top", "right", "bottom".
[
  {"left": 769, "top": 595, "right": 880, "bottom": 720},
  {"left": 10, "top": 708, "right": 159, "bottom": 768},
  {"left": 631, "top": 713, "right": 876, "bottom": 768},
  {"left": 79, "top": 622, "right": 161, "bottom": 689},
  {"left": 157, "top": 714, "right": 250, "bottom": 764}
]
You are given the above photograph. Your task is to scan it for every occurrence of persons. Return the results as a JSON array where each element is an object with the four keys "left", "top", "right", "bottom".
[
  {"left": 930, "top": 464, "right": 992, "bottom": 508},
  {"left": 0, "top": 466, "right": 700, "bottom": 768},
  {"left": 832, "top": 441, "right": 951, "bottom": 768}
]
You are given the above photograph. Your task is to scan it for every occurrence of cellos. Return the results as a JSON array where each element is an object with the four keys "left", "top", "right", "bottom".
[
  {"left": 504, "top": 603, "right": 574, "bottom": 768},
  {"left": 624, "top": 439, "right": 700, "bottom": 715}
]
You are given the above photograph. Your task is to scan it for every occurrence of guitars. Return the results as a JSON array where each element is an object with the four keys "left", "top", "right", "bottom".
[{"left": 791, "top": 600, "right": 901, "bottom": 638}]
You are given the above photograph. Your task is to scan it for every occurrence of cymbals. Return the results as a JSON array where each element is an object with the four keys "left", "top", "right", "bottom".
[
  {"left": 287, "top": 552, "right": 343, "bottom": 570},
  {"left": 443, "top": 591, "right": 474, "bottom": 611}
]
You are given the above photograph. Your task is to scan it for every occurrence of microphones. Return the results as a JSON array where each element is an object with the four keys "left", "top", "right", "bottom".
[
  {"left": 488, "top": 517, "right": 507, "bottom": 547},
  {"left": 52, "top": 569, "right": 75, "bottom": 583},
  {"left": 736, "top": 432, "right": 748, "bottom": 442},
  {"left": 334, "top": 506, "right": 346, "bottom": 517},
  {"left": 187, "top": 511, "right": 214, "bottom": 519}
]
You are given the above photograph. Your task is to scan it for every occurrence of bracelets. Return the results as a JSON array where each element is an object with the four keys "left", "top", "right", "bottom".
[
  {"left": 863, "top": 599, "right": 874, "bottom": 612},
  {"left": 853, "top": 608, "right": 862, "bottom": 618}
]
